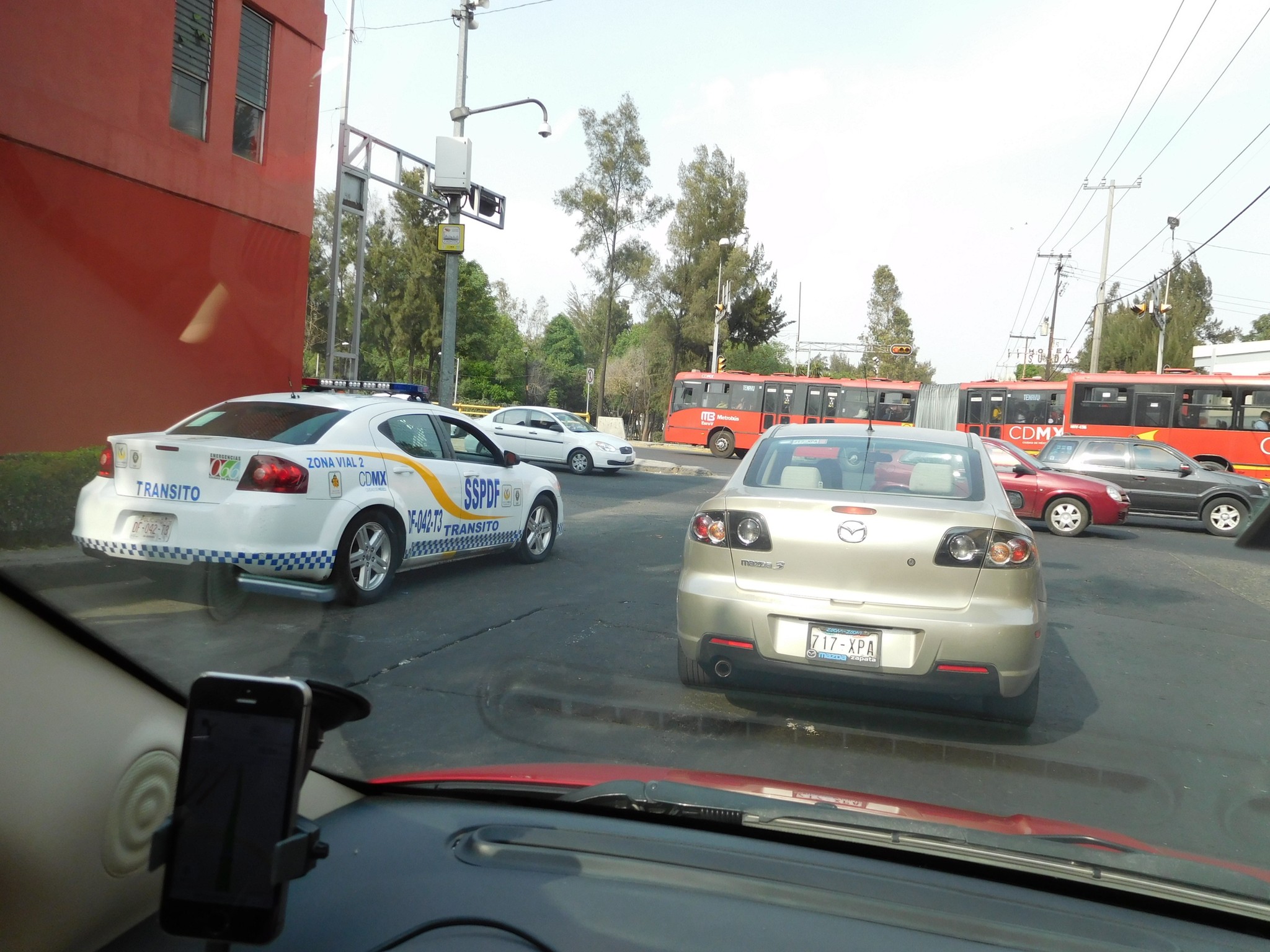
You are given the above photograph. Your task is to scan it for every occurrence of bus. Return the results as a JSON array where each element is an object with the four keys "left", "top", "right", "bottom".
[
  {"left": 1062, "top": 367, "right": 1270, "bottom": 483},
  {"left": 662, "top": 368, "right": 1067, "bottom": 458}
]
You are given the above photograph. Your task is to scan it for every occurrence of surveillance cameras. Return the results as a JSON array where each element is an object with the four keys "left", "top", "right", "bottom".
[{"left": 539, "top": 122, "right": 553, "bottom": 138}]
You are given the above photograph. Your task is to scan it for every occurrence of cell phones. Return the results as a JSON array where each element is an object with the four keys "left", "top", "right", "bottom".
[{"left": 157, "top": 672, "right": 309, "bottom": 941}]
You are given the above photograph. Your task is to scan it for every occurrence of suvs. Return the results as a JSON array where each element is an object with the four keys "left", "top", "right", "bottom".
[{"left": 1035, "top": 433, "right": 1270, "bottom": 538}]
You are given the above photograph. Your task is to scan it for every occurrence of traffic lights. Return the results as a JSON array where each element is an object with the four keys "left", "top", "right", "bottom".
[
  {"left": 713, "top": 303, "right": 722, "bottom": 312},
  {"left": 1161, "top": 304, "right": 1172, "bottom": 314},
  {"left": 717, "top": 357, "right": 726, "bottom": 373},
  {"left": 1129, "top": 303, "right": 1144, "bottom": 315},
  {"left": 890, "top": 344, "right": 913, "bottom": 356}
]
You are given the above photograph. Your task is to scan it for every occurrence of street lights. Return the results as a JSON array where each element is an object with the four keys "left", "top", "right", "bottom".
[
  {"left": 438, "top": 351, "right": 459, "bottom": 404},
  {"left": 521, "top": 346, "right": 530, "bottom": 406},
  {"left": 1155, "top": 216, "right": 1180, "bottom": 374},
  {"left": 711, "top": 237, "right": 730, "bottom": 374},
  {"left": 436, "top": 0, "right": 553, "bottom": 412}
]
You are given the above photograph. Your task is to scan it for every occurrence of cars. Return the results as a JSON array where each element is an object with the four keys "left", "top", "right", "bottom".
[
  {"left": 676, "top": 424, "right": 1047, "bottom": 730},
  {"left": 866, "top": 435, "right": 1132, "bottom": 538},
  {"left": 463, "top": 405, "right": 636, "bottom": 475},
  {"left": 70, "top": 376, "right": 564, "bottom": 610}
]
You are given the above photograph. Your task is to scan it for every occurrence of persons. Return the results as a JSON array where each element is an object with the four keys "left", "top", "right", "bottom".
[
  {"left": 991, "top": 401, "right": 1002, "bottom": 438},
  {"left": 716, "top": 397, "right": 729, "bottom": 408},
  {"left": 735, "top": 396, "right": 746, "bottom": 410},
  {"left": 1007, "top": 397, "right": 1063, "bottom": 425},
  {"left": 1251, "top": 411, "right": 1270, "bottom": 430},
  {"left": 879, "top": 407, "right": 892, "bottom": 421},
  {"left": 815, "top": 458, "right": 843, "bottom": 490}
]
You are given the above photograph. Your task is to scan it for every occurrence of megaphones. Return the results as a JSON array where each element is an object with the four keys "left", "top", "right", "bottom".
[
  {"left": 470, "top": 0, "right": 490, "bottom": 8},
  {"left": 451, "top": 10, "right": 474, "bottom": 21},
  {"left": 467, "top": 19, "right": 478, "bottom": 29}
]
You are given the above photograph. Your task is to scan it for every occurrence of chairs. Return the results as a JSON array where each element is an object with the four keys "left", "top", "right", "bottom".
[
  {"left": 909, "top": 461, "right": 952, "bottom": 496},
  {"left": 780, "top": 466, "right": 822, "bottom": 488}
]
